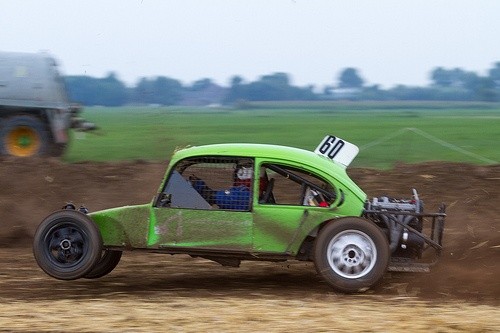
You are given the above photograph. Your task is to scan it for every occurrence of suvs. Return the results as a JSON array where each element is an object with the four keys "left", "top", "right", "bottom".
[{"left": 0, "top": 49, "right": 95, "bottom": 162}]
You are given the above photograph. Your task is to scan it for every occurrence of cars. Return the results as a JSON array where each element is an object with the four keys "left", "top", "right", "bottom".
[{"left": 32, "top": 134, "right": 448, "bottom": 294}]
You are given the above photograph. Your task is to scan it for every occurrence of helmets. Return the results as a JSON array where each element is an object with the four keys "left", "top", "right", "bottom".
[{"left": 236, "top": 160, "right": 265, "bottom": 180}]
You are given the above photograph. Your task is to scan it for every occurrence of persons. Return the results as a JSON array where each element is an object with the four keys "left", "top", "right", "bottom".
[{"left": 190, "top": 159, "right": 254, "bottom": 211}]
